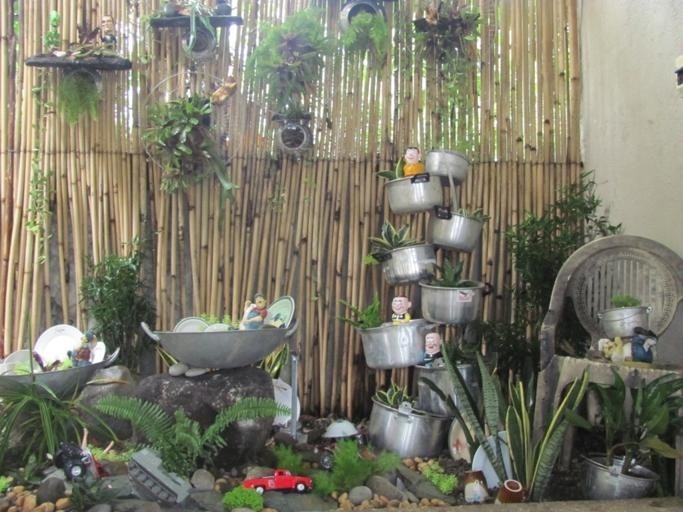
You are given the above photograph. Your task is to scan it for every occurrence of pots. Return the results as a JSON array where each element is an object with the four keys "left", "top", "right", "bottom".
[
  {"left": 426, "top": 150, "right": 469, "bottom": 184},
  {"left": 139, "top": 319, "right": 300, "bottom": 372},
  {"left": 417, "top": 277, "right": 493, "bottom": 324},
  {"left": 373, "top": 243, "right": 436, "bottom": 285},
  {"left": 383, "top": 173, "right": 444, "bottom": 213},
  {"left": 0, "top": 347, "right": 122, "bottom": 395},
  {"left": 351, "top": 317, "right": 440, "bottom": 370},
  {"left": 366, "top": 391, "right": 451, "bottom": 457},
  {"left": 426, "top": 205, "right": 482, "bottom": 253},
  {"left": 598, "top": 306, "right": 652, "bottom": 339},
  {"left": 415, "top": 362, "right": 481, "bottom": 414}
]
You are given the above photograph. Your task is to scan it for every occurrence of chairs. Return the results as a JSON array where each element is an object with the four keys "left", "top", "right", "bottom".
[{"left": 533, "top": 234, "right": 683, "bottom": 501}]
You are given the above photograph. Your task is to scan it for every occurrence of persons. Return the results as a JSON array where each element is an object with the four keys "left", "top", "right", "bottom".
[
  {"left": 596, "top": 326, "right": 661, "bottom": 367},
  {"left": 100, "top": 16, "right": 118, "bottom": 52},
  {"left": 404, "top": 145, "right": 425, "bottom": 175},
  {"left": 56, "top": 330, "right": 93, "bottom": 372},
  {"left": 388, "top": 296, "right": 413, "bottom": 322},
  {"left": 239, "top": 293, "right": 268, "bottom": 330},
  {"left": 424, "top": 333, "right": 444, "bottom": 368},
  {"left": 40, "top": 11, "right": 64, "bottom": 52}
]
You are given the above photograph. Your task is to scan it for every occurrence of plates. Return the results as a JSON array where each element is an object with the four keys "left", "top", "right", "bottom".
[
  {"left": 173, "top": 296, "right": 295, "bottom": 331},
  {"left": 2, "top": 323, "right": 105, "bottom": 372},
  {"left": 444, "top": 407, "right": 515, "bottom": 489},
  {"left": 272, "top": 380, "right": 302, "bottom": 424}
]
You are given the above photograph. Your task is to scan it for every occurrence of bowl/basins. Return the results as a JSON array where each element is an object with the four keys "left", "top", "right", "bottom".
[{"left": 321, "top": 418, "right": 357, "bottom": 439}]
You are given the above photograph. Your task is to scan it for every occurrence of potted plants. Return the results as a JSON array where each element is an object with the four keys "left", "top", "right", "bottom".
[
  {"left": 338, "top": 130, "right": 486, "bottom": 464},
  {"left": 576, "top": 364, "right": 683, "bottom": 502}
]
